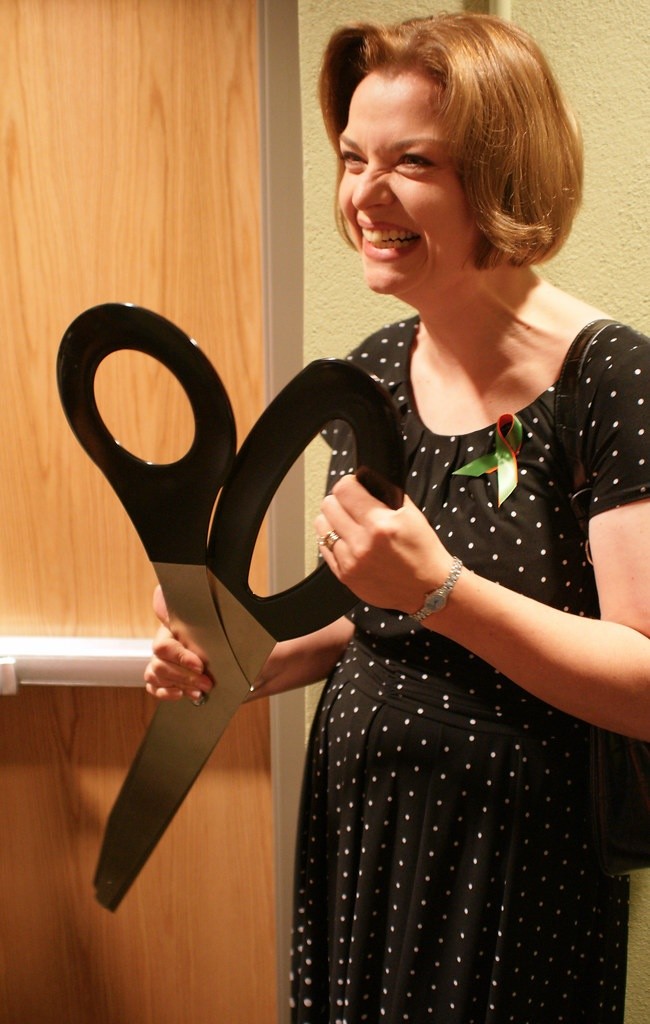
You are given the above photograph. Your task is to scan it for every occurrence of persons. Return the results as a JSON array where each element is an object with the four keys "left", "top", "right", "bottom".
[{"left": 143, "top": 12, "right": 650, "bottom": 1024}]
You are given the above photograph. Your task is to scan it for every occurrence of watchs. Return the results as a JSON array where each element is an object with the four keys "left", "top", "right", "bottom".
[{"left": 409, "top": 555, "right": 463, "bottom": 623}]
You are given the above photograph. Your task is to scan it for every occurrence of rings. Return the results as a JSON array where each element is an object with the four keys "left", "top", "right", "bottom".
[{"left": 316, "top": 529, "right": 340, "bottom": 558}]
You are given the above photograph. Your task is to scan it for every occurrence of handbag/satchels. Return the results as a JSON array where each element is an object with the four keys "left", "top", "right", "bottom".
[{"left": 557, "top": 321, "right": 649, "bottom": 874}]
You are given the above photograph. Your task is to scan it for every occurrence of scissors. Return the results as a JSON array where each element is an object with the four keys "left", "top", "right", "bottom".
[{"left": 53, "top": 301, "right": 408, "bottom": 913}]
